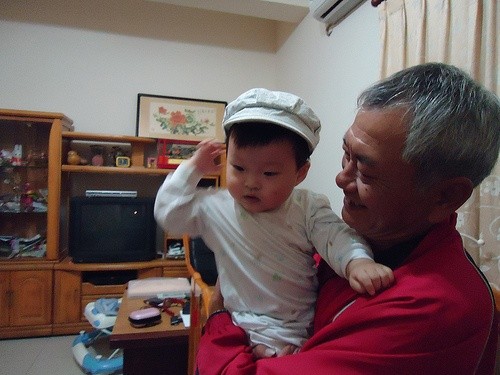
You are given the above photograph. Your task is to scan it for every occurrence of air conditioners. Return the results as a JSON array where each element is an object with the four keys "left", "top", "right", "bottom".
[{"left": 308, "top": 0, "right": 368, "bottom": 37}]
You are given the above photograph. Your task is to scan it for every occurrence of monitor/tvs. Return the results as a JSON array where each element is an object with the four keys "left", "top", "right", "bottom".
[{"left": 68, "top": 197, "right": 156, "bottom": 264}]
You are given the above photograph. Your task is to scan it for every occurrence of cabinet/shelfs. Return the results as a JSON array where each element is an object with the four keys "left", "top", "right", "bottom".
[{"left": 0, "top": 107, "right": 227, "bottom": 340}]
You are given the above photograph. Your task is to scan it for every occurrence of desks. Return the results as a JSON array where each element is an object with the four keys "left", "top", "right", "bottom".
[{"left": 110, "top": 287, "right": 217, "bottom": 375}]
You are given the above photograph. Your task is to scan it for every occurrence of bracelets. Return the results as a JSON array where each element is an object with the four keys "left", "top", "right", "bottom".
[{"left": 206, "top": 308, "right": 227, "bottom": 322}]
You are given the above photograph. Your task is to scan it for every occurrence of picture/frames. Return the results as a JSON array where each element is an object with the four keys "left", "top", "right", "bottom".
[{"left": 134, "top": 93, "right": 229, "bottom": 144}]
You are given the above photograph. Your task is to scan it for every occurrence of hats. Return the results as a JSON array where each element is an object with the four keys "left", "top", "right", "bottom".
[{"left": 223, "top": 88, "right": 322, "bottom": 154}]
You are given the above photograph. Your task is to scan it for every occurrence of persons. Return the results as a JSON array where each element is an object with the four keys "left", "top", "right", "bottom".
[
  {"left": 194, "top": 62, "right": 500, "bottom": 375},
  {"left": 154, "top": 87, "right": 396, "bottom": 359}
]
